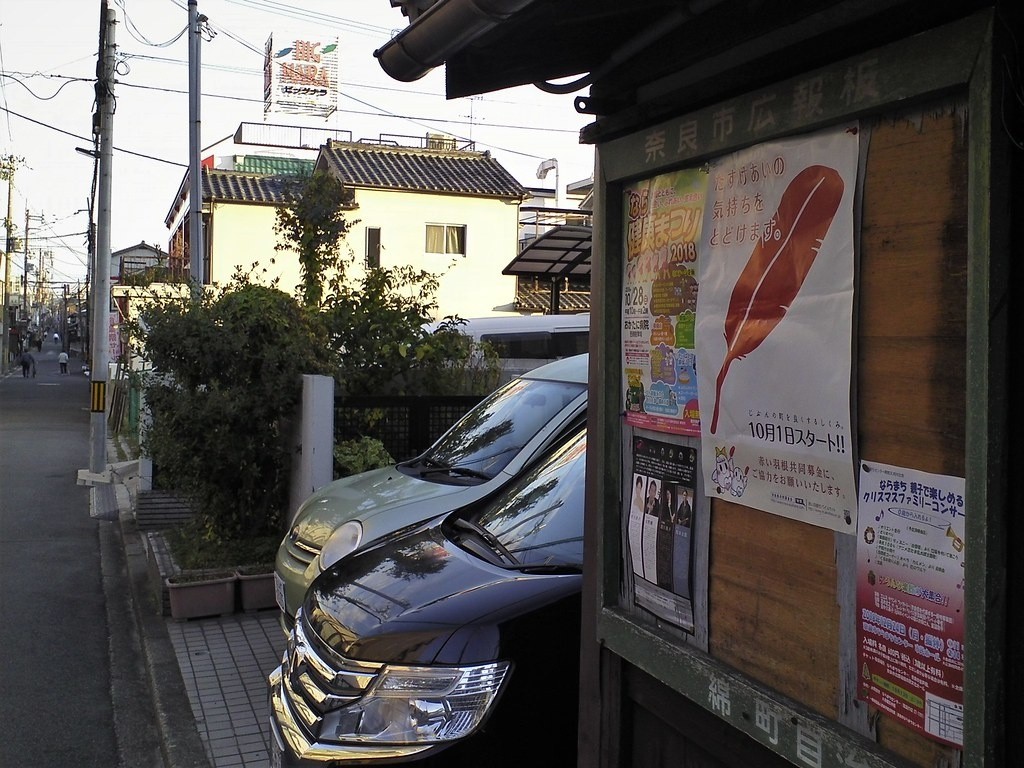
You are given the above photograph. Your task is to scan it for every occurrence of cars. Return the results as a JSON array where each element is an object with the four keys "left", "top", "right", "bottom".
[
  {"left": 275, "top": 352, "right": 593, "bottom": 643},
  {"left": 257, "top": 416, "right": 589, "bottom": 768}
]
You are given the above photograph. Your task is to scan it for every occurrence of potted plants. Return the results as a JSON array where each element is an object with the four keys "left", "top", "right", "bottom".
[{"left": 164, "top": 513, "right": 237, "bottom": 621}]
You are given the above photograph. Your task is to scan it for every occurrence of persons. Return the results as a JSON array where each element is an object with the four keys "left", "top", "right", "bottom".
[
  {"left": 58, "top": 350, "right": 68, "bottom": 375},
  {"left": 645, "top": 481, "right": 659, "bottom": 516},
  {"left": 21, "top": 349, "right": 35, "bottom": 378},
  {"left": 53, "top": 332, "right": 59, "bottom": 344},
  {"left": 632, "top": 476, "right": 643, "bottom": 511},
  {"left": 36, "top": 339, "right": 42, "bottom": 352},
  {"left": 662, "top": 488, "right": 675, "bottom": 522},
  {"left": 677, "top": 490, "right": 691, "bottom": 528}
]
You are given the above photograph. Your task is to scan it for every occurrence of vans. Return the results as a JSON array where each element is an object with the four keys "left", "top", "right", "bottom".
[{"left": 339, "top": 311, "right": 593, "bottom": 407}]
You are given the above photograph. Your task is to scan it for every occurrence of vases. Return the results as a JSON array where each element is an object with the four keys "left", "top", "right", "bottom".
[{"left": 235, "top": 566, "right": 277, "bottom": 613}]
